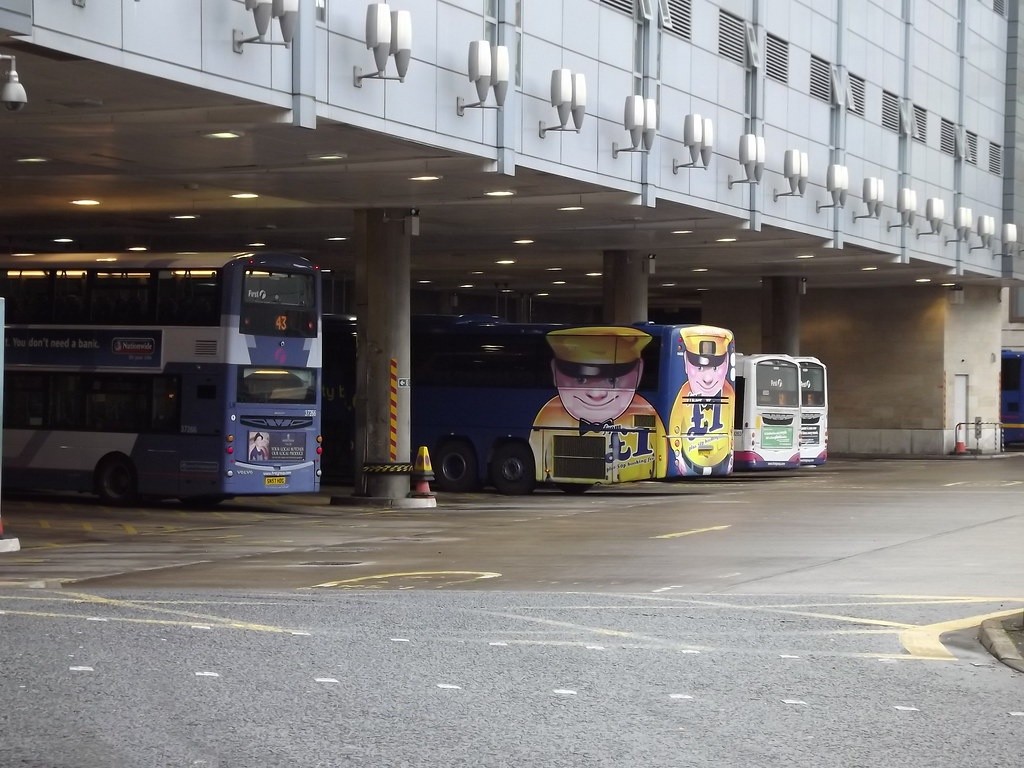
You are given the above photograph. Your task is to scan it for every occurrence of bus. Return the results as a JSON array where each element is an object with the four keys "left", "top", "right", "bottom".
[
  {"left": 321, "top": 312, "right": 736, "bottom": 498},
  {"left": 1000, "top": 349, "right": 1024, "bottom": 450},
  {"left": 794, "top": 356, "right": 828, "bottom": 467},
  {"left": 0, "top": 251, "right": 321, "bottom": 506},
  {"left": 736, "top": 352, "right": 802, "bottom": 472}
]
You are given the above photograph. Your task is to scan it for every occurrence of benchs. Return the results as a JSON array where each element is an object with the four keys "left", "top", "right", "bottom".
[{"left": 9, "top": 294, "right": 211, "bottom": 325}]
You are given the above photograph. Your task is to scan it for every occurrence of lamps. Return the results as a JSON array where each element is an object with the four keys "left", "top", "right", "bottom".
[
  {"left": 644, "top": 254, "right": 656, "bottom": 274},
  {"left": 353, "top": 4, "right": 412, "bottom": 88},
  {"left": 916, "top": 198, "right": 944, "bottom": 239},
  {"left": 949, "top": 286, "right": 965, "bottom": 304},
  {"left": 539, "top": 69, "right": 587, "bottom": 138},
  {"left": 992, "top": 224, "right": 1017, "bottom": 259},
  {"left": 852, "top": 178, "right": 884, "bottom": 223},
  {"left": 455, "top": 40, "right": 510, "bottom": 117},
  {"left": 232, "top": 0, "right": 299, "bottom": 54},
  {"left": 969, "top": 215, "right": 994, "bottom": 253},
  {"left": 611, "top": 96, "right": 657, "bottom": 159},
  {"left": 773, "top": 150, "right": 808, "bottom": 202},
  {"left": 728, "top": 134, "right": 765, "bottom": 189},
  {"left": 816, "top": 165, "right": 848, "bottom": 213},
  {"left": 945, "top": 208, "right": 972, "bottom": 246},
  {"left": 404, "top": 208, "right": 421, "bottom": 236},
  {"left": 673, "top": 114, "right": 713, "bottom": 174},
  {"left": 887, "top": 188, "right": 916, "bottom": 232},
  {"left": 798, "top": 278, "right": 807, "bottom": 295}
]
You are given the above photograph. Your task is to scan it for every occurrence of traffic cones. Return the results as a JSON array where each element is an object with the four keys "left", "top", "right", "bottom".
[{"left": 955, "top": 422, "right": 966, "bottom": 453}]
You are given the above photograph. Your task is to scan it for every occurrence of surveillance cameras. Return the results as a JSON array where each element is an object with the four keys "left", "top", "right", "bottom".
[{"left": 0, "top": 83, "right": 27, "bottom": 112}]
